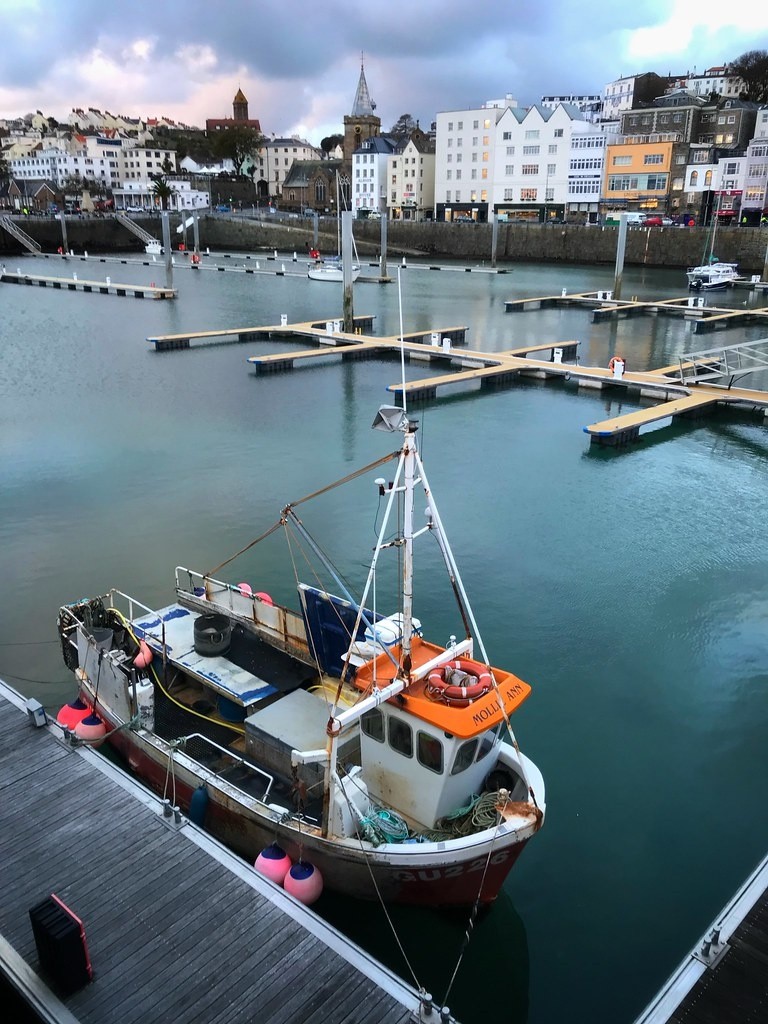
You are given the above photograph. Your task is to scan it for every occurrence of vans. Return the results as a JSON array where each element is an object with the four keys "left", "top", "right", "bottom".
[{"left": 623, "top": 211, "right": 647, "bottom": 227}]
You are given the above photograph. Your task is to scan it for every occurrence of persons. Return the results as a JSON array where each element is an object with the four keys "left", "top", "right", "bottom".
[
  {"left": 2, "top": 263, "right": 7, "bottom": 274},
  {"left": 22, "top": 207, "right": 57, "bottom": 219},
  {"left": 148, "top": 209, "right": 162, "bottom": 220},
  {"left": 77, "top": 206, "right": 104, "bottom": 219},
  {"left": 760, "top": 216, "right": 768, "bottom": 228},
  {"left": 216, "top": 206, "right": 218, "bottom": 213},
  {"left": 115, "top": 206, "right": 125, "bottom": 210},
  {"left": 742, "top": 215, "right": 747, "bottom": 227}
]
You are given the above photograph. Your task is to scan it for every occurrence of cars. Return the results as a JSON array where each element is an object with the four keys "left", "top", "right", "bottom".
[
  {"left": 641, "top": 216, "right": 664, "bottom": 228},
  {"left": 215, "top": 204, "right": 231, "bottom": 213},
  {"left": 455, "top": 215, "right": 475, "bottom": 224},
  {"left": 660, "top": 217, "right": 674, "bottom": 227},
  {"left": 127, "top": 204, "right": 145, "bottom": 214},
  {"left": 547, "top": 216, "right": 568, "bottom": 225}
]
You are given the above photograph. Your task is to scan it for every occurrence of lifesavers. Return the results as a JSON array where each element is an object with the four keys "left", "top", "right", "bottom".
[
  {"left": 427, "top": 686, "right": 493, "bottom": 706},
  {"left": 609, "top": 356, "right": 624, "bottom": 372},
  {"left": 429, "top": 661, "right": 492, "bottom": 699}
]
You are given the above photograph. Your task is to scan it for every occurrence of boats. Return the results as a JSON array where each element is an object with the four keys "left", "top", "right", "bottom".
[{"left": 55, "top": 265, "right": 547, "bottom": 921}]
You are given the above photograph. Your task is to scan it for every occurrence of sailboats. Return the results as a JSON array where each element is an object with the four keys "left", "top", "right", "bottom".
[
  {"left": 307, "top": 167, "right": 362, "bottom": 283},
  {"left": 687, "top": 174, "right": 748, "bottom": 294}
]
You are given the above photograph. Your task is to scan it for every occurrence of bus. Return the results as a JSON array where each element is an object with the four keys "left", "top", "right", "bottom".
[{"left": 497, "top": 208, "right": 541, "bottom": 223}]
